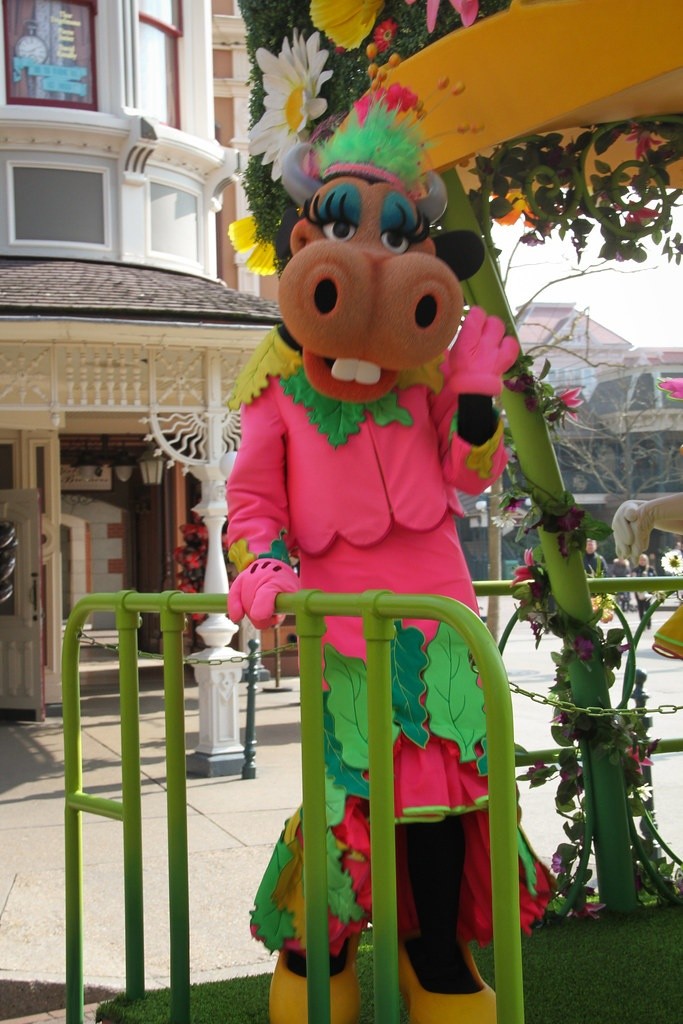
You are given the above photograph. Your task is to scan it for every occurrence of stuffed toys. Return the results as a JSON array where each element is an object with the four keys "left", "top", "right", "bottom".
[
  {"left": 224, "top": 102, "right": 552, "bottom": 1024},
  {"left": 611, "top": 490, "right": 683, "bottom": 563}
]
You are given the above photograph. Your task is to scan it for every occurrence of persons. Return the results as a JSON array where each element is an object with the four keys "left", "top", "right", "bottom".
[
  {"left": 632, "top": 554, "right": 660, "bottom": 630},
  {"left": 612, "top": 555, "right": 632, "bottom": 602},
  {"left": 583, "top": 538, "right": 610, "bottom": 579}
]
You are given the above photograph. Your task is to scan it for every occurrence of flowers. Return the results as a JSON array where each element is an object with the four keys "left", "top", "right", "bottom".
[
  {"left": 176, "top": 512, "right": 226, "bottom": 622},
  {"left": 225, "top": 0, "right": 683, "bottom": 923}
]
